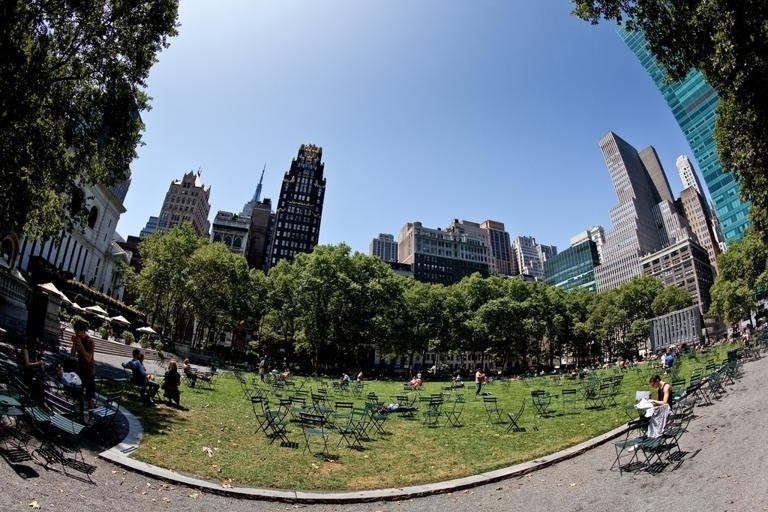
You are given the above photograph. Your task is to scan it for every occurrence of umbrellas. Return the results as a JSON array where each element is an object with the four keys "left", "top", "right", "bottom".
[{"left": 0, "top": 256, "right": 157, "bottom": 338}]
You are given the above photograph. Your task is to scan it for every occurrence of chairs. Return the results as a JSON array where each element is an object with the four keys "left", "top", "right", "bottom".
[
  {"left": 481, "top": 372, "right": 625, "bottom": 435},
  {"left": 233, "top": 368, "right": 391, "bottom": 459},
  {"left": 611, "top": 351, "right": 740, "bottom": 469},
  {"left": 389, "top": 391, "right": 465, "bottom": 428},
  {"left": 1, "top": 374, "right": 125, "bottom": 460}
]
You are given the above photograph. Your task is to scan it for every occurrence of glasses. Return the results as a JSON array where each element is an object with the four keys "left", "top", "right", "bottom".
[{"left": 652, "top": 381, "right": 657, "bottom": 387}]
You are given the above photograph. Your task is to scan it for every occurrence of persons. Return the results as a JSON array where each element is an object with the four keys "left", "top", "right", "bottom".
[
  {"left": 183, "top": 358, "right": 197, "bottom": 388},
  {"left": 474, "top": 366, "right": 486, "bottom": 396},
  {"left": 18, "top": 334, "right": 44, "bottom": 375},
  {"left": 511, "top": 317, "right": 768, "bottom": 380},
  {"left": 404, "top": 371, "right": 423, "bottom": 388},
  {"left": 649, "top": 375, "right": 674, "bottom": 408},
  {"left": 340, "top": 372, "right": 351, "bottom": 388},
  {"left": 378, "top": 402, "right": 420, "bottom": 413},
  {"left": 157, "top": 350, "right": 172, "bottom": 361},
  {"left": 161, "top": 361, "right": 181, "bottom": 406},
  {"left": 451, "top": 373, "right": 465, "bottom": 387},
  {"left": 356, "top": 369, "right": 363, "bottom": 385},
  {"left": 256, "top": 355, "right": 265, "bottom": 382},
  {"left": 121, "top": 347, "right": 160, "bottom": 405},
  {"left": 70, "top": 320, "right": 96, "bottom": 426}
]
